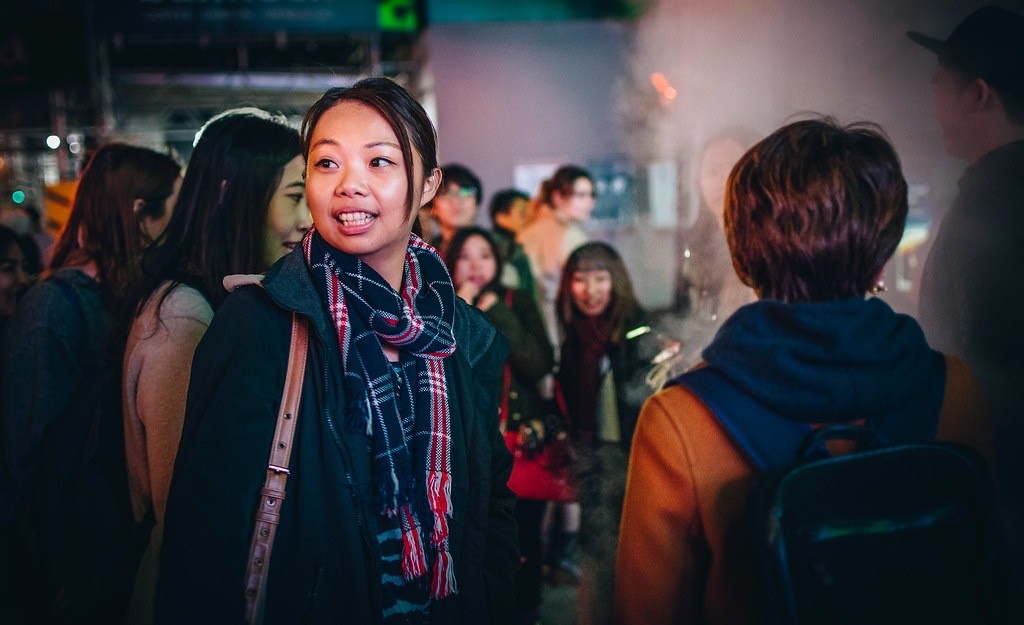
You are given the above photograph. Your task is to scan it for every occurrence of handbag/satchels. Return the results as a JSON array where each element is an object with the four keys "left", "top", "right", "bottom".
[{"left": 500, "top": 430, "right": 583, "bottom": 501}]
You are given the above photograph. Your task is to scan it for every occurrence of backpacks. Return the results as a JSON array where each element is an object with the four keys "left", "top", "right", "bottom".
[{"left": 663, "top": 349, "right": 1024, "bottom": 625}]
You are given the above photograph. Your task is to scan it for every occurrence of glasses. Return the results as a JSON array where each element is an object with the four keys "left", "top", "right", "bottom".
[{"left": 442, "top": 187, "right": 478, "bottom": 199}]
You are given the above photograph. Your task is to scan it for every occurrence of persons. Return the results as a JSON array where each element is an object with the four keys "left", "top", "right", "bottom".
[
  {"left": 423, "top": 159, "right": 689, "bottom": 625},
  {"left": 2, "top": 74, "right": 540, "bottom": 625},
  {"left": 905, "top": 0, "right": 1024, "bottom": 625},
  {"left": 611, "top": 121, "right": 1007, "bottom": 625}
]
